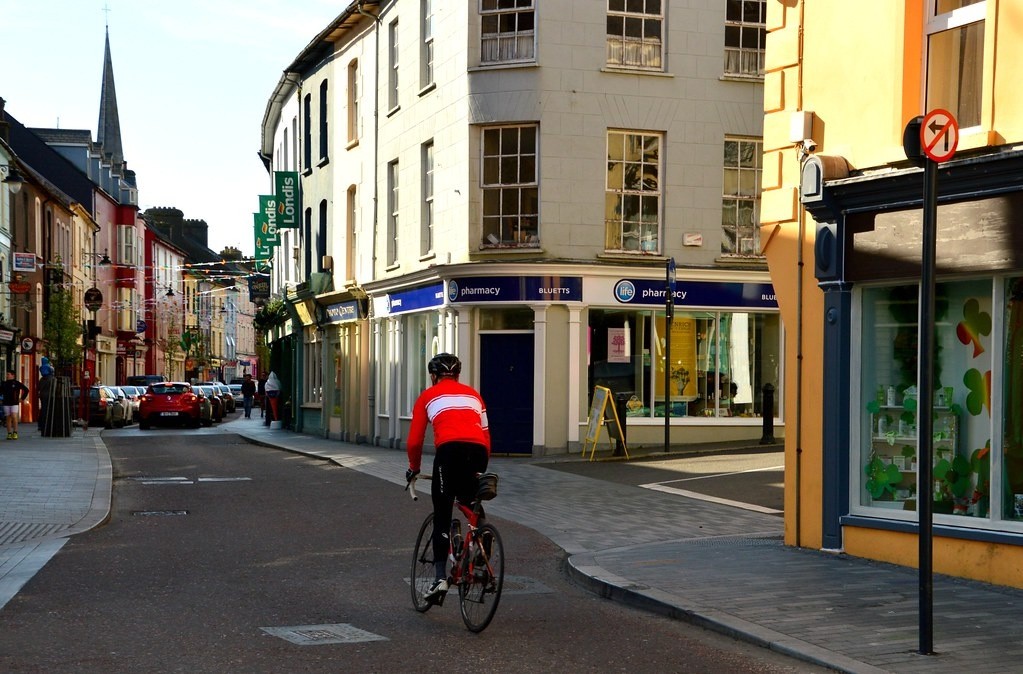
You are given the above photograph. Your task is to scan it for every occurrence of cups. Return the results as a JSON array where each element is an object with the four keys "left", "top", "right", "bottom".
[{"left": 704, "top": 407, "right": 713, "bottom": 417}]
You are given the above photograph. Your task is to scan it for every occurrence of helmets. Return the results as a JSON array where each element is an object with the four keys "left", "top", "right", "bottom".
[{"left": 429, "top": 353, "right": 461, "bottom": 375}]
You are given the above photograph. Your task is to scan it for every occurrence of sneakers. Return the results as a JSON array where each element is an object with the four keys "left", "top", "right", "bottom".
[
  {"left": 476, "top": 531, "right": 493, "bottom": 567},
  {"left": 13, "top": 432, "right": 18, "bottom": 439},
  {"left": 424, "top": 578, "right": 448, "bottom": 600},
  {"left": 7, "top": 433, "right": 14, "bottom": 440}
]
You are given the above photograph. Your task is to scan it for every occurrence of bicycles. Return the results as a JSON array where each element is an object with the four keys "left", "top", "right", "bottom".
[{"left": 405, "top": 470, "right": 506, "bottom": 633}]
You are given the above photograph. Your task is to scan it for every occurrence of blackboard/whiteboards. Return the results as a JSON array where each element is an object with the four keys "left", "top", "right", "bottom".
[{"left": 584, "top": 384, "right": 625, "bottom": 443}]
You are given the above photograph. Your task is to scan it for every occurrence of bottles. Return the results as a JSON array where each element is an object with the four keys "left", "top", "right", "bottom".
[
  {"left": 910, "top": 455, "right": 916, "bottom": 471},
  {"left": 887, "top": 385, "right": 895, "bottom": 406},
  {"left": 449, "top": 519, "right": 462, "bottom": 546},
  {"left": 898, "top": 417, "right": 907, "bottom": 435},
  {"left": 934, "top": 479, "right": 942, "bottom": 499},
  {"left": 939, "top": 394, "right": 944, "bottom": 407},
  {"left": 878, "top": 414, "right": 887, "bottom": 437},
  {"left": 877, "top": 384, "right": 886, "bottom": 405},
  {"left": 933, "top": 456, "right": 936, "bottom": 469}
]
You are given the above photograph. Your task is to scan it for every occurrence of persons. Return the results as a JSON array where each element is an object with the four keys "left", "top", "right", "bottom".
[
  {"left": 241, "top": 370, "right": 269, "bottom": 419},
  {"left": 38, "top": 357, "right": 54, "bottom": 431},
  {"left": 406, "top": 353, "right": 490, "bottom": 602},
  {"left": 93, "top": 377, "right": 102, "bottom": 386},
  {"left": 0, "top": 370, "right": 29, "bottom": 440}
]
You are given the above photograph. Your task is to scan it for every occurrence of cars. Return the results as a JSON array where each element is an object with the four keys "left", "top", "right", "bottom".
[{"left": 70, "top": 379, "right": 259, "bottom": 430}]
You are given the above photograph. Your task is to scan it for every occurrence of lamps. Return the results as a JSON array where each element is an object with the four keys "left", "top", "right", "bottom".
[
  {"left": 211, "top": 303, "right": 226, "bottom": 313},
  {"left": 0, "top": 163, "right": 29, "bottom": 194},
  {"left": 165, "top": 285, "right": 176, "bottom": 299},
  {"left": 83, "top": 249, "right": 111, "bottom": 266}
]
